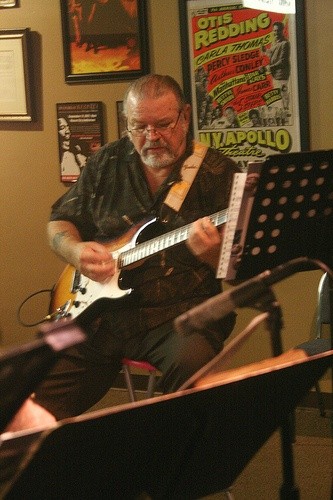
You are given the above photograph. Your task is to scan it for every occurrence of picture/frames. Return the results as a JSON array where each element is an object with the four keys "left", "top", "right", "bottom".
[
  {"left": 0, "top": 27, "right": 35, "bottom": 124},
  {"left": 56, "top": 101, "right": 105, "bottom": 184},
  {"left": 177, "top": 0, "right": 311, "bottom": 173},
  {"left": 60, "top": 0, "right": 151, "bottom": 85},
  {"left": 116, "top": 99, "right": 128, "bottom": 139}
]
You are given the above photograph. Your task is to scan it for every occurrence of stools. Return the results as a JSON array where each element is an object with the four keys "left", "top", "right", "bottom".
[{"left": 122, "top": 359, "right": 158, "bottom": 403}]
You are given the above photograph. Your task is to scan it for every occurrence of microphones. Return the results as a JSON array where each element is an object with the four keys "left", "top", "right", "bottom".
[{"left": 175, "top": 256, "right": 308, "bottom": 336}]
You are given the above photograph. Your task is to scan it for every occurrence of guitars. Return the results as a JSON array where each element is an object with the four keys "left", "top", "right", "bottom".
[{"left": 48, "top": 208, "right": 230, "bottom": 328}]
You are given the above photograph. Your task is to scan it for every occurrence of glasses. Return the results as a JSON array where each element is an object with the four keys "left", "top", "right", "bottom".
[{"left": 125, "top": 108, "right": 181, "bottom": 137}]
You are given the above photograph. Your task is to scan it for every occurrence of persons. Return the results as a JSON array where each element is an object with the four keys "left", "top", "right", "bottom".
[
  {"left": 3, "top": 73, "right": 246, "bottom": 439},
  {"left": 58, "top": 116, "right": 89, "bottom": 178},
  {"left": 195, "top": 21, "right": 293, "bottom": 130}
]
